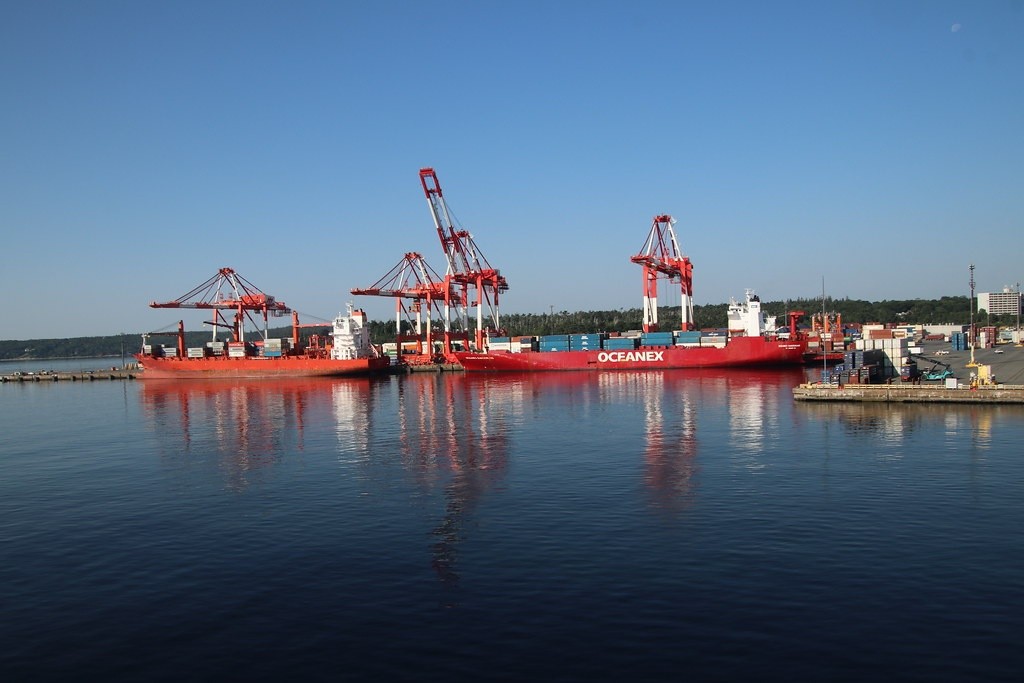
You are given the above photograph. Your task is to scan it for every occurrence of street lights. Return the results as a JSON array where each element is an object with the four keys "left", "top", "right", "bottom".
[
  {"left": 1016, "top": 282, "right": 1021, "bottom": 346},
  {"left": 968, "top": 264, "right": 976, "bottom": 366}
]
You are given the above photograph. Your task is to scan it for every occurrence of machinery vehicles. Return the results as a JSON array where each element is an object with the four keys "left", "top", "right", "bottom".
[{"left": 907, "top": 350, "right": 953, "bottom": 381}]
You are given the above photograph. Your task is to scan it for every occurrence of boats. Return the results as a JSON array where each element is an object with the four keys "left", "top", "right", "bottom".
[
  {"left": 132, "top": 311, "right": 390, "bottom": 375},
  {"left": 452, "top": 291, "right": 808, "bottom": 370}
]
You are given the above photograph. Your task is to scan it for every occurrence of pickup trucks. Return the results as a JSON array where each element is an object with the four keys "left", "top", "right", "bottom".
[
  {"left": 994, "top": 349, "right": 1003, "bottom": 354},
  {"left": 937, "top": 350, "right": 949, "bottom": 355}
]
